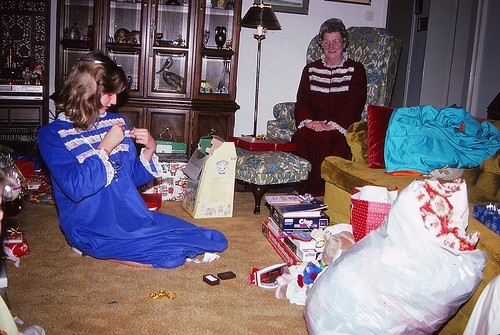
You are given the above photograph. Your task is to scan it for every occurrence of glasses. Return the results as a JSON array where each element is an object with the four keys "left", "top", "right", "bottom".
[{"left": 320, "top": 38, "right": 344, "bottom": 48}]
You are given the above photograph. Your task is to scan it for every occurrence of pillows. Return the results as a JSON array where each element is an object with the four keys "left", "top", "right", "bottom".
[{"left": 368, "top": 104, "right": 394, "bottom": 169}]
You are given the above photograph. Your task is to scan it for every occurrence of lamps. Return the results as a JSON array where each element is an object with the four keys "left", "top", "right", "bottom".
[{"left": 240, "top": 4, "right": 282, "bottom": 137}]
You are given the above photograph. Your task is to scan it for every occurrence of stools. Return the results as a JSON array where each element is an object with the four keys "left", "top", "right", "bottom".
[{"left": 235, "top": 146, "right": 312, "bottom": 216}]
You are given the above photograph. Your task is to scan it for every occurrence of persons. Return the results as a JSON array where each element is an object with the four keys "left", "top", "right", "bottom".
[
  {"left": 38, "top": 51, "right": 228, "bottom": 269},
  {"left": 292, "top": 18, "right": 367, "bottom": 197}
]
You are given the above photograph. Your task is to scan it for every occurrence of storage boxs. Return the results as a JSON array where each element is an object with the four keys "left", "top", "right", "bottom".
[
  {"left": 261, "top": 205, "right": 330, "bottom": 266},
  {"left": 0, "top": 266, "right": 8, "bottom": 288},
  {"left": 156, "top": 140, "right": 187, "bottom": 153},
  {"left": 229, "top": 137, "right": 297, "bottom": 151}
]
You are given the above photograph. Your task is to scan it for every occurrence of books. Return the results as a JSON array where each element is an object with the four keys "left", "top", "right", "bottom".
[{"left": 266, "top": 196, "right": 331, "bottom": 262}]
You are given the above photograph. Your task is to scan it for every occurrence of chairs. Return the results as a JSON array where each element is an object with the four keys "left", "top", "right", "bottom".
[{"left": 269, "top": 26, "right": 402, "bottom": 142}]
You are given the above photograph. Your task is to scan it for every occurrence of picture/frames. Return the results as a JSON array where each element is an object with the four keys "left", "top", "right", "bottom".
[{"left": 254, "top": 0, "right": 371, "bottom": 15}]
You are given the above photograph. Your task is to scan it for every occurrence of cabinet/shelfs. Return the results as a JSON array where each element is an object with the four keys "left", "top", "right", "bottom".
[
  {"left": 56, "top": 0, "right": 242, "bottom": 159},
  {"left": 0, "top": 105, "right": 43, "bottom": 128}
]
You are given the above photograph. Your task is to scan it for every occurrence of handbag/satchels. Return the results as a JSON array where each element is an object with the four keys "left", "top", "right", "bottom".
[{"left": 350, "top": 185, "right": 400, "bottom": 242}]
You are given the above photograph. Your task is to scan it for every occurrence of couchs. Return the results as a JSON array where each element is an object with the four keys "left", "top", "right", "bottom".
[{"left": 321, "top": 121, "right": 500, "bottom": 335}]
